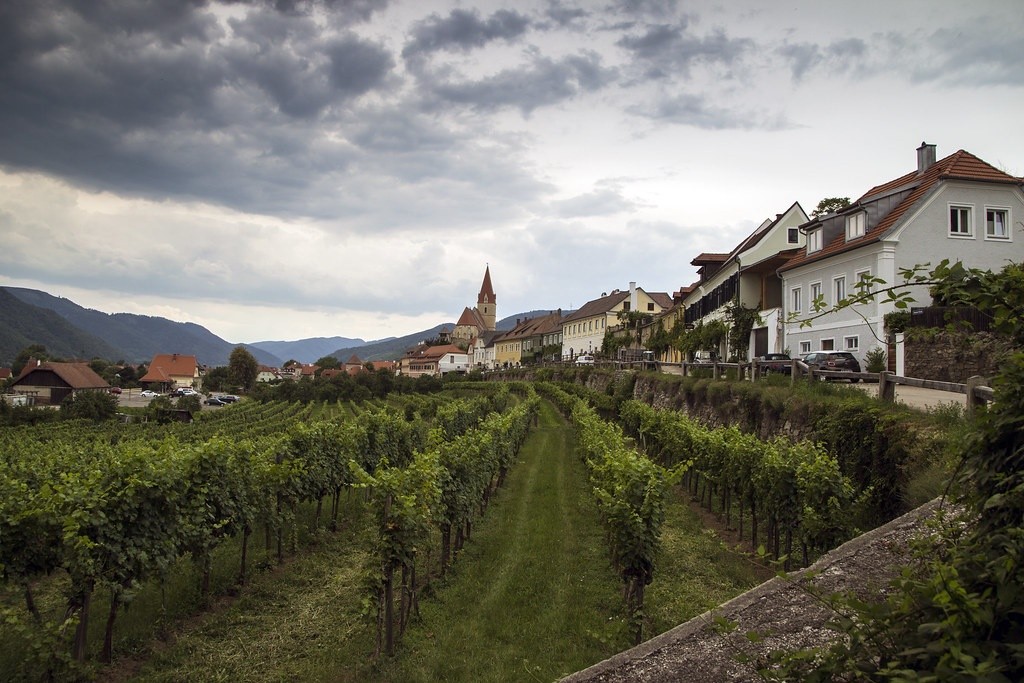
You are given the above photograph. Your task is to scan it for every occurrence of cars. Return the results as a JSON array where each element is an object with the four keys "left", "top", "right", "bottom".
[
  {"left": 748, "top": 352, "right": 792, "bottom": 376},
  {"left": 694, "top": 350, "right": 723, "bottom": 365},
  {"left": 204, "top": 394, "right": 241, "bottom": 406},
  {"left": 168, "top": 386, "right": 198, "bottom": 397},
  {"left": 801, "top": 350, "right": 861, "bottom": 383},
  {"left": 139, "top": 390, "right": 160, "bottom": 398}
]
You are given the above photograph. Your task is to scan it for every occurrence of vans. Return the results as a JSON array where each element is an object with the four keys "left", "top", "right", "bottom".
[{"left": 574, "top": 355, "right": 595, "bottom": 366}]
